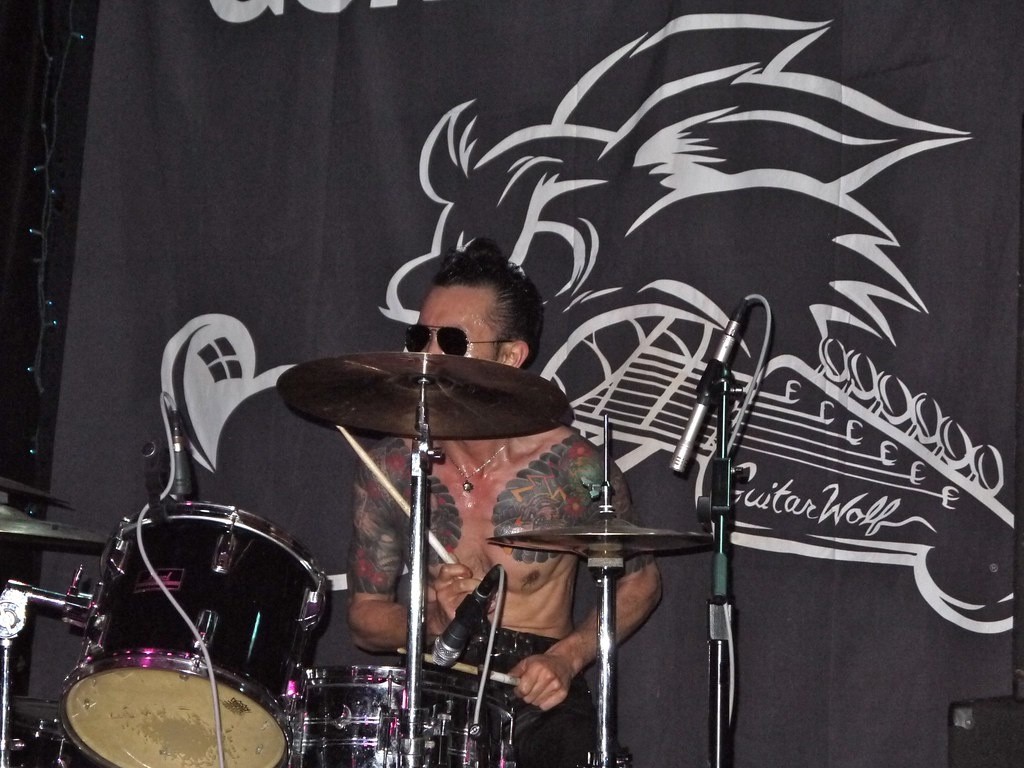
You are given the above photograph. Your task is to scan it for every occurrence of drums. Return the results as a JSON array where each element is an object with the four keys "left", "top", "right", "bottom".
[
  {"left": 58, "top": 498, "right": 331, "bottom": 767},
  {"left": 289, "top": 666, "right": 518, "bottom": 768}
]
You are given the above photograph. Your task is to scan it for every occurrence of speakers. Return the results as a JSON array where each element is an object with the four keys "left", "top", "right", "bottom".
[{"left": 947, "top": 696, "right": 1024, "bottom": 768}]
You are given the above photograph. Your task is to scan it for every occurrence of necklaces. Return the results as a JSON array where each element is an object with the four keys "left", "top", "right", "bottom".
[{"left": 439, "top": 441, "right": 508, "bottom": 491}]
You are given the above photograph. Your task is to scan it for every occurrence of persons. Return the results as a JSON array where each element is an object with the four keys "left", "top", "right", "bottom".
[{"left": 345, "top": 238, "right": 660, "bottom": 767}]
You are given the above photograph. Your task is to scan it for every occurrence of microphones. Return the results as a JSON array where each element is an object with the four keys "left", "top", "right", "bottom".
[
  {"left": 668, "top": 299, "right": 750, "bottom": 474},
  {"left": 169, "top": 410, "right": 193, "bottom": 495},
  {"left": 432, "top": 564, "right": 501, "bottom": 666}
]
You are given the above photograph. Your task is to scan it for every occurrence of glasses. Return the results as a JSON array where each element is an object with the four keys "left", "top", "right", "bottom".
[{"left": 403, "top": 323, "right": 516, "bottom": 357}]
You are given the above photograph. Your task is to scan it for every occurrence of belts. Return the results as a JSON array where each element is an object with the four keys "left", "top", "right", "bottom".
[{"left": 464, "top": 629, "right": 550, "bottom": 657}]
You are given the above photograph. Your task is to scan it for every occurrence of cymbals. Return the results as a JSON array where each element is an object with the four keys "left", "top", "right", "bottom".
[
  {"left": 0, "top": 505, "right": 110, "bottom": 544},
  {"left": 485, "top": 517, "right": 716, "bottom": 559},
  {"left": 277, "top": 351, "right": 574, "bottom": 437}
]
